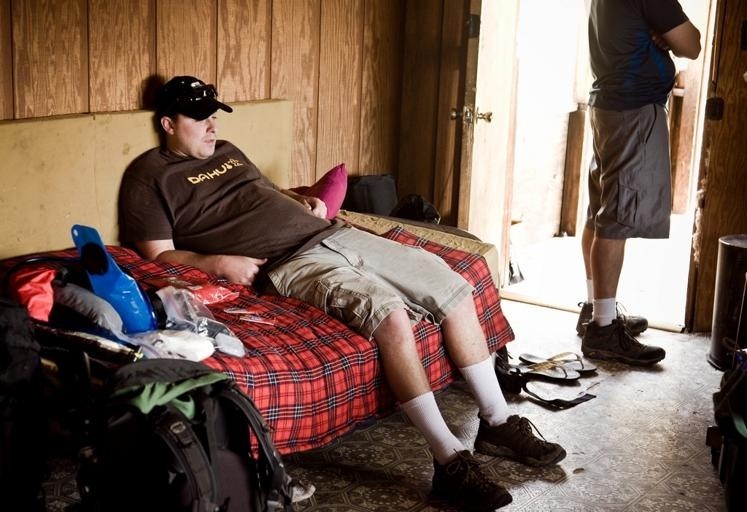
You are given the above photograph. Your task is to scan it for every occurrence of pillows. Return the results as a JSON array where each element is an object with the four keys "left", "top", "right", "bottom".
[{"left": 288, "top": 163, "right": 350, "bottom": 221}]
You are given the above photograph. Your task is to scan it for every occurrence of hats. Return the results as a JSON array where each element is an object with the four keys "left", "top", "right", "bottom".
[{"left": 163, "top": 75, "right": 233, "bottom": 120}]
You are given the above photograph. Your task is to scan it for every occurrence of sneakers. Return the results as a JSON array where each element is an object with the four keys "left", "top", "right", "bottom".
[
  {"left": 582, "top": 320, "right": 665, "bottom": 366},
  {"left": 431, "top": 449, "right": 512, "bottom": 510},
  {"left": 475, "top": 414, "right": 566, "bottom": 466},
  {"left": 575, "top": 301, "right": 648, "bottom": 335}
]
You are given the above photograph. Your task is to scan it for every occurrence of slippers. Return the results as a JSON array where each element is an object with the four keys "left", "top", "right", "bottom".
[{"left": 507, "top": 352, "right": 597, "bottom": 382}]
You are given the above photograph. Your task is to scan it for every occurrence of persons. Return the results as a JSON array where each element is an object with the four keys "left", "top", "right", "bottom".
[
  {"left": 121, "top": 75, "right": 567, "bottom": 512},
  {"left": 577, "top": 0, "right": 702, "bottom": 367}
]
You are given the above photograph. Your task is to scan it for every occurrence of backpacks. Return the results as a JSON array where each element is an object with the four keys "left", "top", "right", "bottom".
[{"left": 102, "top": 358, "right": 293, "bottom": 512}]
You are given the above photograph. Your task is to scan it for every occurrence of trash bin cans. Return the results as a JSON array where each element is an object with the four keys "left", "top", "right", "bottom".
[{"left": 706, "top": 234, "right": 747, "bottom": 373}]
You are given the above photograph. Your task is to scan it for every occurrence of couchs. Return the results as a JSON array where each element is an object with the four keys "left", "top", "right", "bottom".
[{"left": 0, "top": 95, "right": 515, "bottom": 460}]
